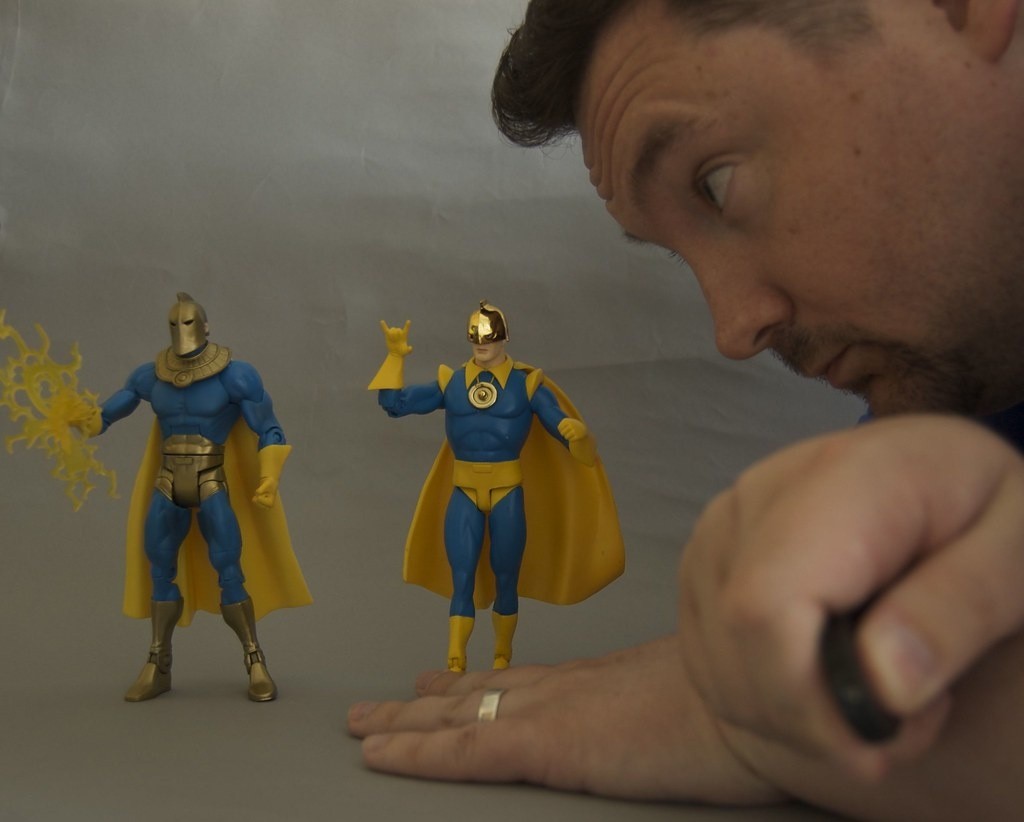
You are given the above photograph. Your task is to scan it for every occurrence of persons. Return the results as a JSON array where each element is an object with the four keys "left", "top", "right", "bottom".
[
  {"left": 366, "top": 298, "right": 625, "bottom": 672},
  {"left": 348, "top": 0, "right": 1023, "bottom": 822},
  {"left": 54, "top": 292, "right": 314, "bottom": 701}
]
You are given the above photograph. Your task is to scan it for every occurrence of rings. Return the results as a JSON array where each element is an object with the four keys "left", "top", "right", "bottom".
[{"left": 478, "top": 687, "right": 510, "bottom": 721}]
